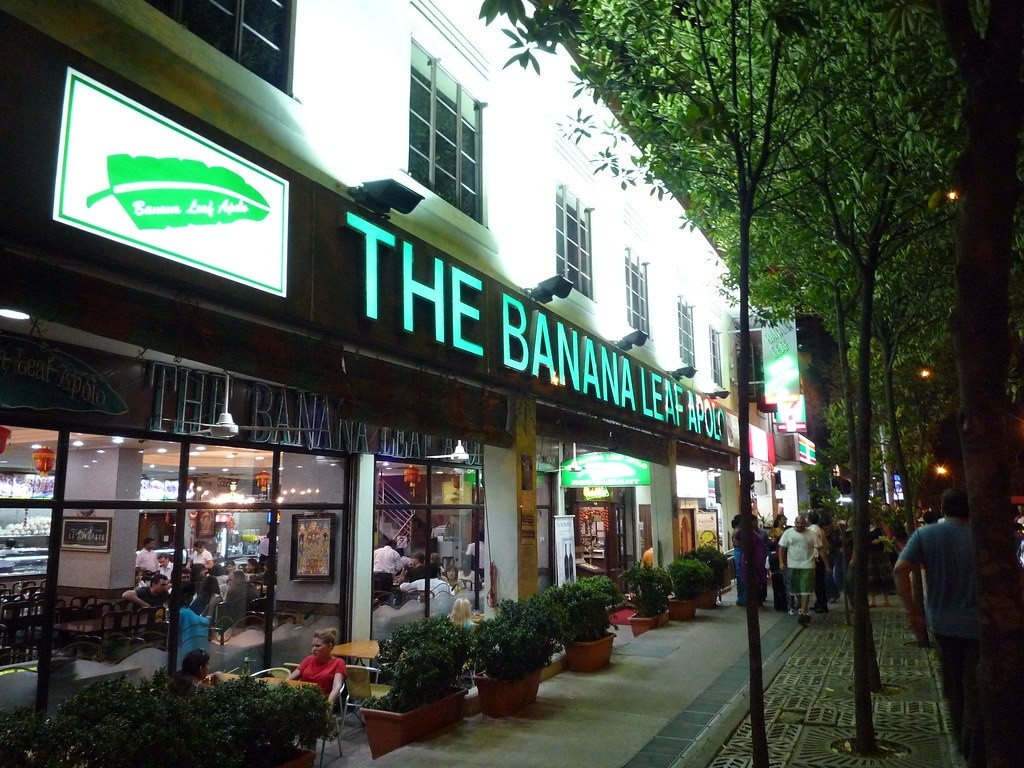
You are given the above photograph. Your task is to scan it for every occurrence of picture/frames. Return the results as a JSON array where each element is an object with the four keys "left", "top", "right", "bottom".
[
  {"left": 59, "top": 517, "right": 112, "bottom": 552},
  {"left": 291, "top": 513, "right": 335, "bottom": 583}
]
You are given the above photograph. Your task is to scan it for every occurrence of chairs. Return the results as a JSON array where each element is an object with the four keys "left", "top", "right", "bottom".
[
  {"left": 0, "top": 579, "right": 169, "bottom": 665},
  {"left": 283, "top": 663, "right": 300, "bottom": 670},
  {"left": 339, "top": 665, "right": 395, "bottom": 739},
  {"left": 318, "top": 682, "right": 345, "bottom": 768},
  {"left": 250, "top": 667, "right": 291, "bottom": 681}
]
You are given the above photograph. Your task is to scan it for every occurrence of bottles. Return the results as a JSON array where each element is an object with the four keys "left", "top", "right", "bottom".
[{"left": 241, "top": 657, "right": 251, "bottom": 678}]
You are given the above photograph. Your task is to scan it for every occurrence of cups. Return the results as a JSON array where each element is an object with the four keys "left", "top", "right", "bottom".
[{"left": 473, "top": 609, "right": 481, "bottom": 617}]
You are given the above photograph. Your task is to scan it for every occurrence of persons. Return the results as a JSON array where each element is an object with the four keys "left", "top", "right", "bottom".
[
  {"left": 894, "top": 488, "right": 998, "bottom": 757},
  {"left": 465, "top": 531, "right": 486, "bottom": 580},
  {"left": 172, "top": 581, "right": 224, "bottom": 670},
  {"left": 680, "top": 515, "right": 690, "bottom": 555},
  {"left": 257, "top": 527, "right": 271, "bottom": 562},
  {"left": 168, "top": 649, "right": 210, "bottom": 698},
  {"left": 373, "top": 540, "right": 483, "bottom": 601},
  {"left": 1011, "top": 504, "right": 1024, "bottom": 567},
  {"left": 283, "top": 627, "right": 348, "bottom": 707},
  {"left": 122, "top": 539, "right": 279, "bottom": 628},
  {"left": 640, "top": 540, "right": 661, "bottom": 570},
  {"left": 636, "top": 516, "right": 660, "bottom": 545},
  {"left": 731, "top": 502, "right": 949, "bottom": 626},
  {"left": 450, "top": 598, "right": 476, "bottom": 632}
]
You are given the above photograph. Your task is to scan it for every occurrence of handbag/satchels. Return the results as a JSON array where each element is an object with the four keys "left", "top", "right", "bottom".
[{"left": 872, "top": 544, "right": 893, "bottom": 575}]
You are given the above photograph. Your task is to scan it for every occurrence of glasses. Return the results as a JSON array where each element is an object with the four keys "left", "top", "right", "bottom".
[{"left": 198, "top": 647, "right": 208, "bottom": 656}]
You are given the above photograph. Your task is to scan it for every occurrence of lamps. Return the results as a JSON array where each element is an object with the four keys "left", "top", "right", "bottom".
[
  {"left": 529, "top": 273, "right": 575, "bottom": 307},
  {"left": 708, "top": 390, "right": 731, "bottom": 401},
  {"left": 349, "top": 179, "right": 426, "bottom": 223},
  {"left": 671, "top": 367, "right": 696, "bottom": 381},
  {"left": 617, "top": 328, "right": 648, "bottom": 352}
]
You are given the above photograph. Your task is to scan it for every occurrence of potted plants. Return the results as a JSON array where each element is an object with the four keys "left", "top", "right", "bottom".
[
  {"left": 538, "top": 573, "right": 628, "bottom": 674},
  {"left": 664, "top": 558, "right": 714, "bottom": 622},
  {"left": 617, "top": 559, "right": 678, "bottom": 637},
  {"left": 462, "top": 592, "right": 581, "bottom": 718},
  {"left": 684, "top": 543, "right": 729, "bottom": 610},
  {"left": 358, "top": 613, "right": 478, "bottom": 760},
  {"left": 0, "top": 669, "right": 341, "bottom": 768}
]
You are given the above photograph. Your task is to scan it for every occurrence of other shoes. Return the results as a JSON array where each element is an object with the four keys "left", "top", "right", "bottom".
[
  {"left": 815, "top": 605, "right": 829, "bottom": 613},
  {"left": 809, "top": 603, "right": 819, "bottom": 610},
  {"left": 798, "top": 608, "right": 803, "bottom": 615},
  {"left": 788, "top": 608, "right": 795, "bottom": 615}
]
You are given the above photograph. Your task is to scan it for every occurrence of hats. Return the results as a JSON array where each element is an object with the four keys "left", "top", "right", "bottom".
[{"left": 846, "top": 516, "right": 853, "bottom": 531}]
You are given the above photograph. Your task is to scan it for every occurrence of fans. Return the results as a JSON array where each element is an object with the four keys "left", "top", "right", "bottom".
[
  {"left": 162, "top": 373, "right": 327, "bottom": 436},
  {"left": 544, "top": 442, "right": 604, "bottom": 474},
  {"left": 425, "top": 439, "right": 486, "bottom": 462}
]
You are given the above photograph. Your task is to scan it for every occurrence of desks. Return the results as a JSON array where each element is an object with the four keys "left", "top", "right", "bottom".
[
  {"left": 206, "top": 673, "right": 318, "bottom": 687},
  {"left": 0, "top": 581, "right": 46, "bottom": 590},
  {"left": 2, "top": 605, "right": 43, "bottom": 628},
  {"left": 310, "top": 640, "right": 406, "bottom": 667},
  {"left": 53, "top": 614, "right": 163, "bottom": 633}
]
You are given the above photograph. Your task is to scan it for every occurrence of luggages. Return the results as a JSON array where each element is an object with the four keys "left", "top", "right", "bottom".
[{"left": 799, "top": 614, "right": 811, "bottom": 623}]
